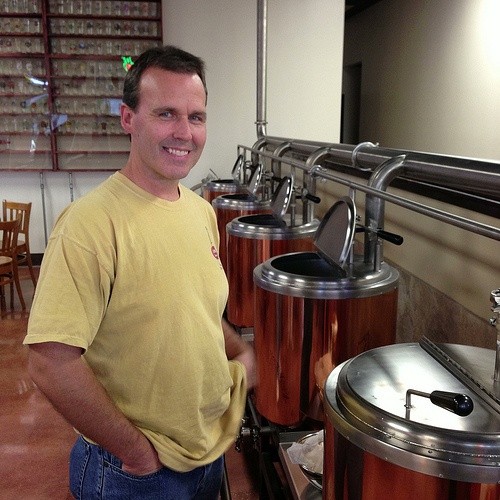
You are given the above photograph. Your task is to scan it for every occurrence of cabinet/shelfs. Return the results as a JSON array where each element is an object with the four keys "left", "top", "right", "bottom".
[{"left": 0, "top": 0, "right": 163, "bottom": 173}]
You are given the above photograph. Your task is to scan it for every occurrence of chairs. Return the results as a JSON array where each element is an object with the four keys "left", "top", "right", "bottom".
[{"left": 0, "top": 200, "right": 38, "bottom": 319}]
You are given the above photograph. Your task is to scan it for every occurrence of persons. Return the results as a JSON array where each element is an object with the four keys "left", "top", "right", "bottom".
[{"left": 22, "top": 45, "right": 256, "bottom": 500}]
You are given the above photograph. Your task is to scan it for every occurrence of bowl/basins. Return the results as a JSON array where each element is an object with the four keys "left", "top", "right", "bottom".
[{"left": 296, "top": 433, "right": 322, "bottom": 490}]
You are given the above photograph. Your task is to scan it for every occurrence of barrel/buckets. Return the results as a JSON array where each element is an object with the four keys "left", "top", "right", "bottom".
[
  {"left": 227, "top": 213, "right": 320, "bottom": 327},
  {"left": 322, "top": 343, "right": 499, "bottom": 500},
  {"left": 211, "top": 193, "right": 273, "bottom": 277},
  {"left": 254, "top": 251, "right": 400, "bottom": 427},
  {"left": 202, "top": 178, "right": 259, "bottom": 205}
]
